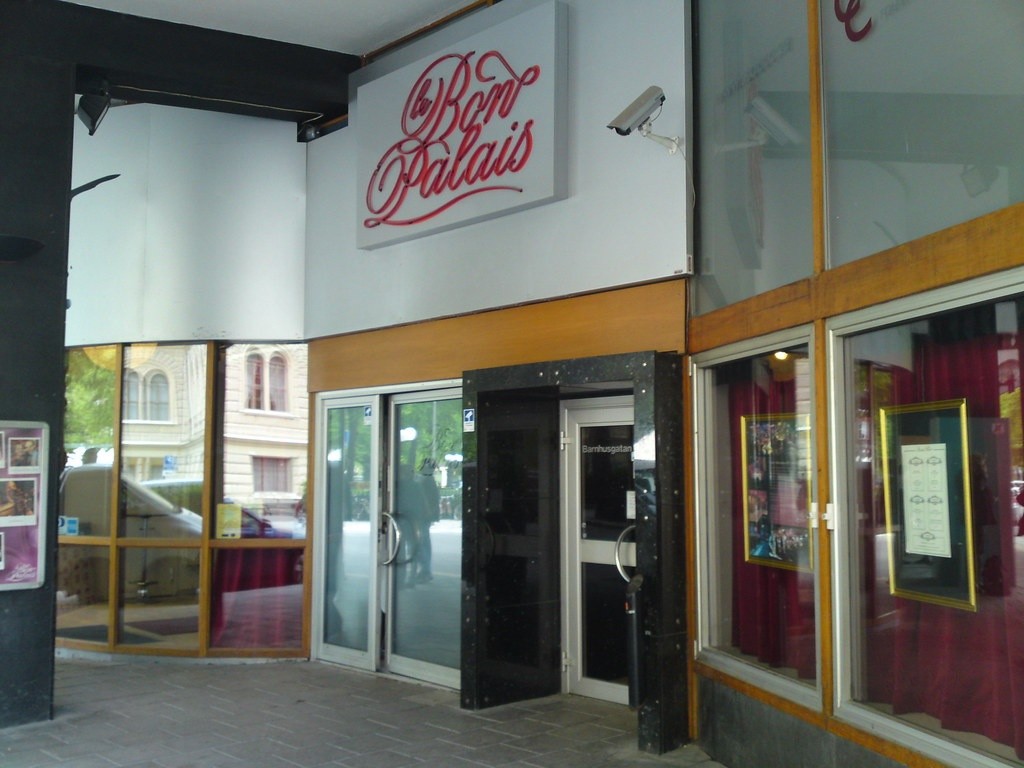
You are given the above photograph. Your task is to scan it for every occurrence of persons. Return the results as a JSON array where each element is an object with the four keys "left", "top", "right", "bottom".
[
  {"left": 6, "top": 482, "right": 34, "bottom": 516},
  {"left": 392, "top": 461, "right": 440, "bottom": 590},
  {"left": 11, "top": 440, "right": 38, "bottom": 466}
]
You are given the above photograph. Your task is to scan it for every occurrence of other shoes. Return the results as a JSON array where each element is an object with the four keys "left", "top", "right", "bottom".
[
  {"left": 394, "top": 576, "right": 404, "bottom": 588},
  {"left": 405, "top": 578, "right": 415, "bottom": 588},
  {"left": 416, "top": 570, "right": 435, "bottom": 583}
]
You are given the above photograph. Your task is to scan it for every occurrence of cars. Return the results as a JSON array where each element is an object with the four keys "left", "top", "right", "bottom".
[{"left": 56, "top": 460, "right": 307, "bottom": 606}]
